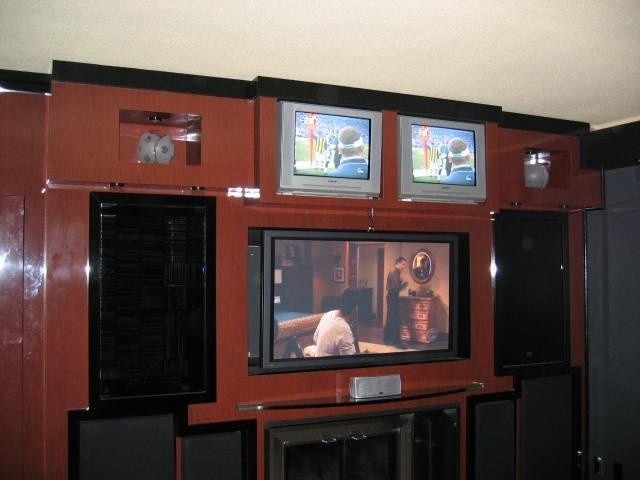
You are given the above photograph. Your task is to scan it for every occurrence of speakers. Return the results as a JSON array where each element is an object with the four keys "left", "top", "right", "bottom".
[
  {"left": 351, "top": 375, "right": 404, "bottom": 397},
  {"left": 249, "top": 247, "right": 262, "bottom": 365}
]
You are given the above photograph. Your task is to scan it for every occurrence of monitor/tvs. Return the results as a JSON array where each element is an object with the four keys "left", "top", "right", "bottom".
[
  {"left": 400, "top": 115, "right": 488, "bottom": 204},
  {"left": 281, "top": 100, "right": 381, "bottom": 199},
  {"left": 262, "top": 230, "right": 458, "bottom": 371}
]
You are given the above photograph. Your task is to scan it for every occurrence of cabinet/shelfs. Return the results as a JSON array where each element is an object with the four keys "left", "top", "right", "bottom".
[
  {"left": 467, "top": 368, "right": 582, "bottom": 480},
  {"left": 69, "top": 409, "right": 256, "bottom": 480},
  {"left": 267, "top": 417, "right": 408, "bottom": 480},
  {"left": 47, "top": 60, "right": 257, "bottom": 190},
  {"left": 497, "top": 123, "right": 583, "bottom": 208}
]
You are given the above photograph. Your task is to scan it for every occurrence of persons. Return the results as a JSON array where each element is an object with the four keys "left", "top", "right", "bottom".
[
  {"left": 438, "top": 136, "right": 476, "bottom": 188},
  {"left": 324, "top": 125, "right": 370, "bottom": 180},
  {"left": 313, "top": 287, "right": 361, "bottom": 358},
  {"left": 382, "top": 256, "right": 408, "bottom": 348}
]
